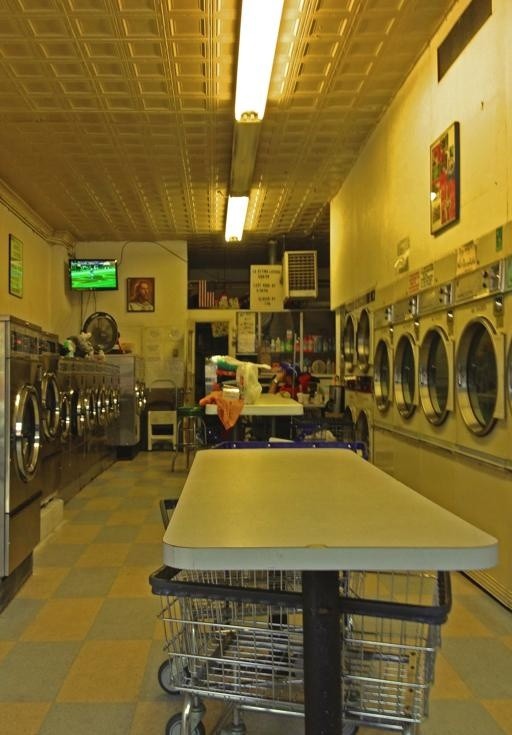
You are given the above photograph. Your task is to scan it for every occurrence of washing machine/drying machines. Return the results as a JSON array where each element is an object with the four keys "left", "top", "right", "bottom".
[
  {"left": 330, "top": 221, "right": 511, "bottom": 613},
  {"left": 1, "top": 315, "right": 146, "bottom": 578}
]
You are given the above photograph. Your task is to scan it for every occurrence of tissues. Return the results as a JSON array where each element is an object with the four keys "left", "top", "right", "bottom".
[{"left": 221, "top": 383, "right": 242, "bottom": 401}]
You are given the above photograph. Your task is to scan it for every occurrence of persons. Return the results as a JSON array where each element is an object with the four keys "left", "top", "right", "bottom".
[
  {"left": 128, "top": 281, "right": 153, "bottom": 311},
  {"left": 272, "top": 362, "right": 281, "bottom": 370},
  {"left": 70, "top": 260, "right": 111, "bottom": 279},
  {"left": 269, "top": 368, "right": 312, "bottom": 401}
]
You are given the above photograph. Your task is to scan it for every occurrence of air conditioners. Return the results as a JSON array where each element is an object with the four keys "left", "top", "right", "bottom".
[{"left": 282, "top": 249, "right": 319, "bottom": 304}]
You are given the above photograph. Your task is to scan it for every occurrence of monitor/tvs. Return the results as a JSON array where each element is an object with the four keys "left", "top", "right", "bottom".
[{"left": 69, "top": 259, "right": 118, "bottom": 290}]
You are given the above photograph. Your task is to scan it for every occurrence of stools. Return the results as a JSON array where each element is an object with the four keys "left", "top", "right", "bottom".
[{"left": 171, "top": 404, "right": 208, "bottom": 475}]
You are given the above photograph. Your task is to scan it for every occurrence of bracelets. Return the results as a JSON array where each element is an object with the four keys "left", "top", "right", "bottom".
[{"left": 272, "top": 378, "right": 279, "bottom": 384}]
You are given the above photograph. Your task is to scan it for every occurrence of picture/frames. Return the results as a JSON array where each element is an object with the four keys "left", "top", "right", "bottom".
[
  {"left": 429, "top": 120, "right": 461, "bottom": 235},
  {"left": 126, "top": 277, "right": 155, "bottom": 313}
]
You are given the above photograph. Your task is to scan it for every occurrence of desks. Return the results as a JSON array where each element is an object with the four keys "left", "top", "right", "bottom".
[
  {"left": 204, "top": 390, "right": 305, "bottom": 444},
  {"left": 163, "top": 448, "right": 498, "bottom": 735}
]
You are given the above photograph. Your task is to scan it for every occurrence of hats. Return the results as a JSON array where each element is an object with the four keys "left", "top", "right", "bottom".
[{"left": 279, "top": 362, "right": 302, "bottom": 380}]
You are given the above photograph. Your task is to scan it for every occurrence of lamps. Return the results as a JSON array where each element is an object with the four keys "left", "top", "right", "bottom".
[
  {"left": 224, "top": 194, "right": 249, "bottom": 242},
  {"left": 229, "top": 121, "right": 260, "bottom": 197},
  {"left": 234, "top": 0, "right": 285, "bottom": 122}
]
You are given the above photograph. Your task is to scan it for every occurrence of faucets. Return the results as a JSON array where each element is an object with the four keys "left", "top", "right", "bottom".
[{"left": 149, "top": 378, "right": 178, "bottom": 410}]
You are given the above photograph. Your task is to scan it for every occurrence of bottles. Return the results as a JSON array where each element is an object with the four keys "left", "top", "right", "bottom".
[
  {"left": 272, "top": 357, "right": 335, "bottom": 375},
  {"left": 264, "top": 331, "right": 335, "bottom": 351}
]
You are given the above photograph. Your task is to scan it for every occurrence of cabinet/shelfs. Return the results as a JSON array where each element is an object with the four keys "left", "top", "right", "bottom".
[{"left": 235, "top": 309, "right": 337, "bottom": 410}]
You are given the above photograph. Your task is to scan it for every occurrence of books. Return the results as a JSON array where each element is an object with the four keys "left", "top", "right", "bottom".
[{"left": 223, "top": 384, "right": 240, "bottom": 400}]
